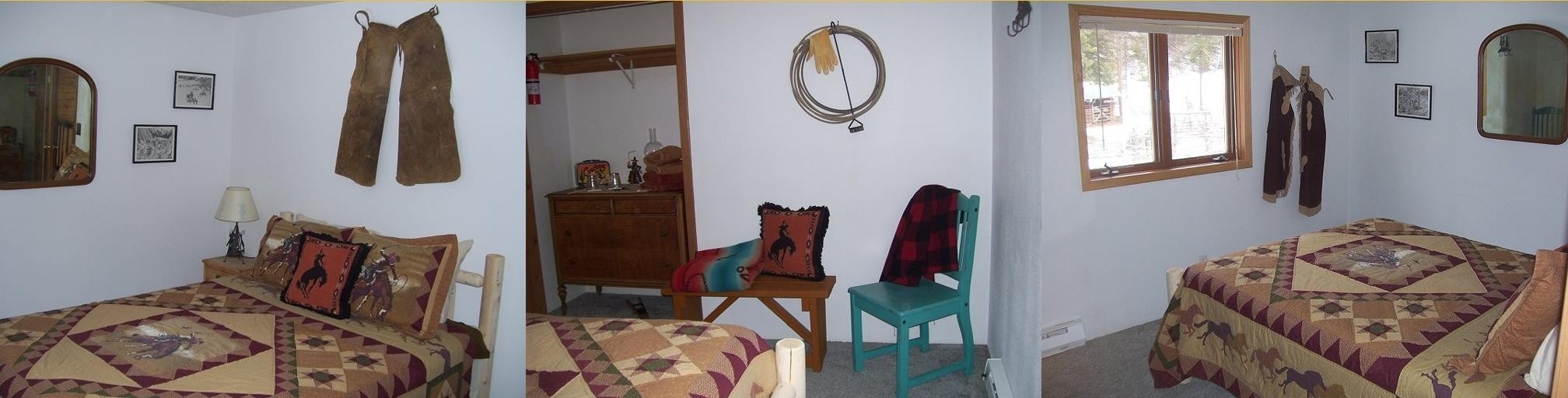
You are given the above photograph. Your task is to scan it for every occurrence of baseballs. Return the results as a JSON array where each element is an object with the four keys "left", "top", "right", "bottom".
[{"left": 526, "top": 50, "right": 543, "bottom": 104}]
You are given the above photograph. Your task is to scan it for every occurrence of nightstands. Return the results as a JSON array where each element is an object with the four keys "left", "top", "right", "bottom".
[{"left": 202, "top": 256, "right": 257, "bottom": 283}]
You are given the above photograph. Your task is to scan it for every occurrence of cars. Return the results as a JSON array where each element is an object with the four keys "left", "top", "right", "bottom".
[{"left": 585, "top": 174, "right": 601, "bottom": 192}]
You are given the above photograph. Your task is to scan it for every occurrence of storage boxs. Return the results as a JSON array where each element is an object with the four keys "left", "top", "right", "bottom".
[{"left": 661, "top": 275, "right": 837, "bottom": 373}]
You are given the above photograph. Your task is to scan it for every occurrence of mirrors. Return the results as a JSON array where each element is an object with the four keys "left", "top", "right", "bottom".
[
  {"left": 1478, "top": 25, "right": 1567, "bottom": 144},
  {"left": 0, "top": 58, "right": 97, "bottom": 191}
]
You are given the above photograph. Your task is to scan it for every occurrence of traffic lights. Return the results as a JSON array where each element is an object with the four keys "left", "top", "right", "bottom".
[
  {"left": 758, "top": 202, "right": 830, "bottom": 282},
  {"left": 52, "top": 143, "right": 89, "bottom": 180},
  {"left": 1472, "top": 243, "right": 1568, "bottom": 397}
]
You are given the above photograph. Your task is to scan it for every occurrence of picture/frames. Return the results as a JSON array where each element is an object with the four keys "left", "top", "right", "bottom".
[
  {"left": 1365, "top": 30, "right": 1433, "bottom": 121},
  {"left": 132, "top": 71, "right": 216, "bottom": 164}
]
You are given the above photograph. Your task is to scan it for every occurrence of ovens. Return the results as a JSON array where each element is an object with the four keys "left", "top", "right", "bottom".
[
  {"left": 2, "top": 211, "right": 506, "bottom": 398},
  {"left": 1167, "top": 218, "right": 1568, "bottom": 397},
  {"left": 527, "top": 315, "right": 807, "bottom": 398}
]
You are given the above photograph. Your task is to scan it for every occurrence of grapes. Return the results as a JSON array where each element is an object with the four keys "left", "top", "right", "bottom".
[{"left": 202, "top": 256, "right": 257, "bottom": 283}]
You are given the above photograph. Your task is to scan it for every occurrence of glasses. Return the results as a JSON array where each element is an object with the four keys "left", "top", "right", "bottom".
[{"left": 214, "top": 186, "right": 260, "bottom": 265}]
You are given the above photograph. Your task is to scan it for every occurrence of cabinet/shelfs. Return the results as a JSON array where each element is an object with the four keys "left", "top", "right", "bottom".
[
  {"left": 547, "top": 196, "right": 687, "bottom": 316},
  {"left": 607, "top": 172, "right": 622, "bottom": 191},
  {"left": 644, "top": 128, "right": 664, "bottom": 156}
]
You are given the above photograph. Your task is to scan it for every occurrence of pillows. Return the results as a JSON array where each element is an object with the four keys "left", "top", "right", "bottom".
[
  {"left": 758, "top": 202, "right": 830, "bottom": 282},
  {"left": 1472, "top": 243, "right": 1568, "bottom": 397},
  {"left": 253, "top": 215, "right": 458, "bottom": 341},
  {"left": 52, "top": 143, "right": 89, "bottom": 180}
]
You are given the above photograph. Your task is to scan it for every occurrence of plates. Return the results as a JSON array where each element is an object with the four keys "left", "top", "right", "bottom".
[{"left": 547, "top": 196, "right": 687, "bottom": 316}]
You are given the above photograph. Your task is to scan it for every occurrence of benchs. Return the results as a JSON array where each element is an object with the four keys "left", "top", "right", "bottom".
[{"left": 661, "top": 275, "right": 837, "bottom": 373}]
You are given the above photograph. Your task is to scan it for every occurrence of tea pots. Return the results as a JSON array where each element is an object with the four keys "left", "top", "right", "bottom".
[
  {"left": 0, "top": 58, "right": 97, "bottom": 191},
  {"left": 1478, "top": 25, "right": 1567, "bottom": 144}
]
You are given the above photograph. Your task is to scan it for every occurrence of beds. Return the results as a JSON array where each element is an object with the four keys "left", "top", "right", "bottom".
[
  {"left": 2, "top": 211, "right": 506, "bottom": 398},
  {"left": 527, "top": 315, "right": 807, "bottom": 398},
  {"left": 1167, "top": 218, "right": 1568, "bottom": 397}
]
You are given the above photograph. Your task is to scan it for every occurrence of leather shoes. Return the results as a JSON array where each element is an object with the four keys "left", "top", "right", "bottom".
[{"left": 806, "top": 30, "right": 839, "bottom": 75}]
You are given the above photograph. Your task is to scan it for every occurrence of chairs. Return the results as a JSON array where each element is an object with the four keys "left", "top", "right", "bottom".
[
  {"left": 848, "top": 186, "right": 980, "bottom": 398},
  {"left": 1532, "top": 107, "right": 1564, "bottom": 139}
]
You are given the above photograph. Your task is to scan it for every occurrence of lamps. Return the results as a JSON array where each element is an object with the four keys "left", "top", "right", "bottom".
[
  {"left": 848, "top": 186, "right": 980, "bottom": 398},
  {"left": 214, "top": 186, "right": 260, "bottom": 265},
  {"left": 1532, "top": 107, "right": 1564, "bottom": 139}
]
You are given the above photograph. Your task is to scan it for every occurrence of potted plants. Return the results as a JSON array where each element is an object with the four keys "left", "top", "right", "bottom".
[
  {"left": 1365, "top": 30, "right": 1433, "bottom": 121},
  {"left": 132, "top": 71, "right": 216, "bottom": 164}
]
